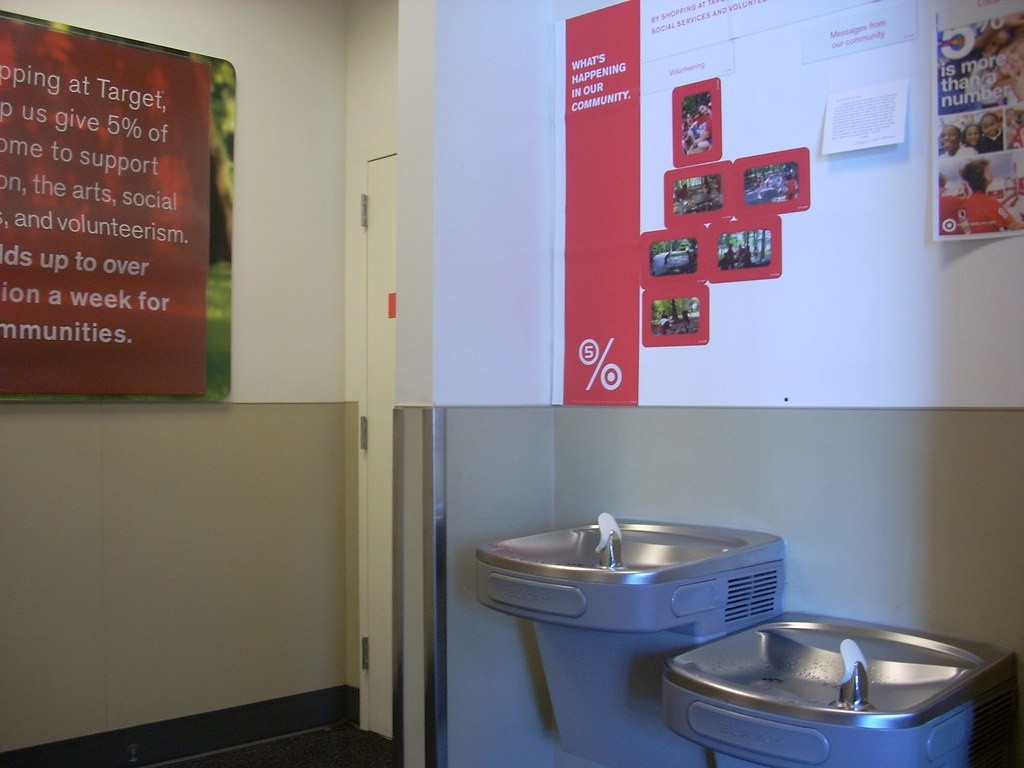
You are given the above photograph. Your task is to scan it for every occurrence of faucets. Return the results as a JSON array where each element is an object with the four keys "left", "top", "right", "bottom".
[
  {"left": 829, "top": 636, "right": 874, "bottom": 713},
  {"left": 592, "top": 511, "right": 627, "bottom": 570}
]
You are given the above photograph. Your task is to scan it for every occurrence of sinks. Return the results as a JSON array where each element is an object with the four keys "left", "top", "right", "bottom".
[
  {"left": 661, "top": 609, "right": 1019, "bottom": 768},
  {"left": 473, "top": 515, "right": 786, "bottom": 631}
]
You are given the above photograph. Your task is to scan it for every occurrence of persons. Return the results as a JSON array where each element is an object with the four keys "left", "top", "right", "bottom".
[
  {"left": 686, "top": 246, "right": 697, "bottom": 270},
  {"left": 682, "top": 103, "right": 712, "bottom": 154},
  {"left": 718, "top": 244, "right": 752, "bottom": 270},
  {"left": 939, "top": 12, "right": 1024, "bottom": 235},
  {"left": 678, "top": 177, "right": 721, "bottom": 215},
  {"left": 755, "top": 168, "right": 797, "bottom": 201},
  {"left": 657, "top": 309, "right": 691, "bottom": 335},
  {"left": 664, "top": 252, "right": 670, "bottom": 265}
]
